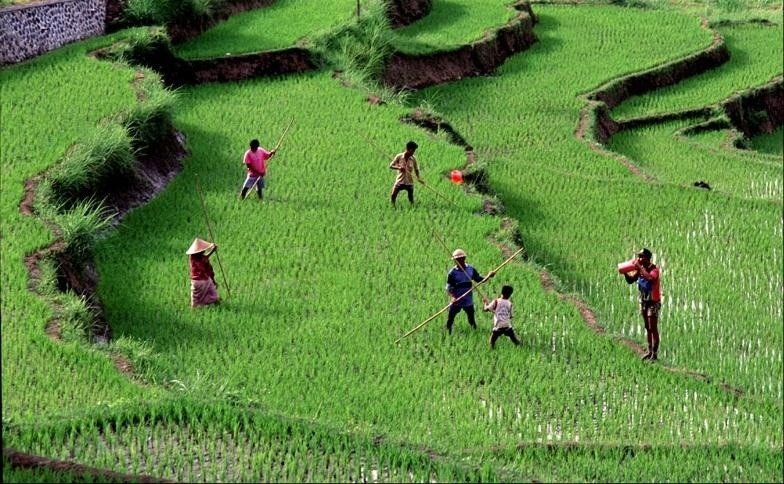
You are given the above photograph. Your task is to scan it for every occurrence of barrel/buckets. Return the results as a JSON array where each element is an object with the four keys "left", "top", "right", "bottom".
[
  {"left": 617, "top": 259, "right": 638, "bottom": 274},
  {"left": 450, "top": 170, "right": 463, "bottom": 183}
]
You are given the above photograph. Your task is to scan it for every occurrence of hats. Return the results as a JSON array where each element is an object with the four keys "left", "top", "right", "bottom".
[
  {"left": 635, "top": 248, "right": 652, "bottom": 258},
  {"left": 186, "top": 238, "right": 214, "bottom": 255},
  {"left": 450, "top": 249, "right": 466, "bottom": 260}
]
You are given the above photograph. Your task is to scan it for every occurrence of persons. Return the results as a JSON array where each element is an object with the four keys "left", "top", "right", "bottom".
[
  {"left": 444, "top": 247, "right": 496, "bottom": 333},
  {"left": 184, "top": 237, "right": 220, "bottom": 305},
  {"left": 387, "top": 140, "right": 426, "bottom": 205},
  {"left": 616, "top": 247, "right": 661, "bottom": 361},
  {"left": 481, "top": 284, "right": 522, "bottom": 348},
  {"left": 238, "top": 138, "right": 275, "bottom": 200}
]
